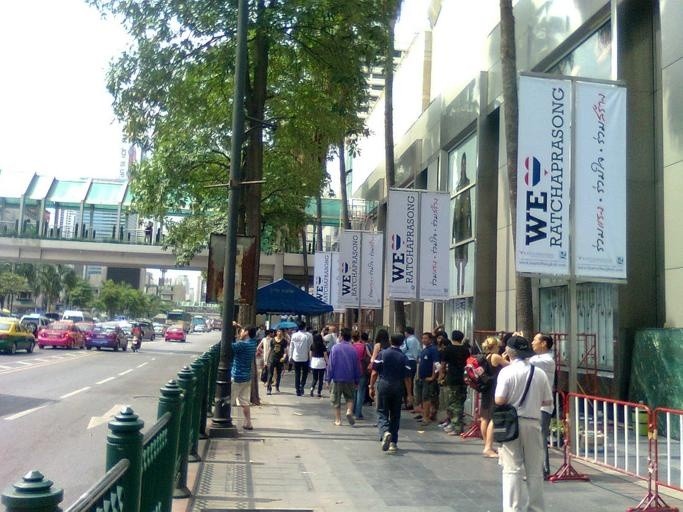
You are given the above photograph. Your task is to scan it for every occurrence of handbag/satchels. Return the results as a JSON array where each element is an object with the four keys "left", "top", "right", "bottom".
[
  {"left": 492, "top": 403, "right": 518, "bottom": 442},
  {"left": 261, "top": 366, "right": 268, "bottom": 382}
]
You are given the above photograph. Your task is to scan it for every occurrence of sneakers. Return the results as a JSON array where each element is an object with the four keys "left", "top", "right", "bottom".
[
  {"left": 410, "top": 407, "right": 436, "bottom": 425},
  {"left": 437, "top": 417, "right": 456, "bottom": 436},
  {"left": 346, "top": 414, "right": 355, "bottom": 425},
  {"left": 381, "top": 433, "right": 398, "bottom": 452}
]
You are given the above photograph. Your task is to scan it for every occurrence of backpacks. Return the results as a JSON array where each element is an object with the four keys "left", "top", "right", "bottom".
[{"left": 464, "top": 352, "right": 495, "bottom": 391}]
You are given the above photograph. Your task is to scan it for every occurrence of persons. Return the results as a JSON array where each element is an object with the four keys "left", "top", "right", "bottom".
[
  {"left": 131, "top": 323, "right": 143, "bottom": 339},
  {"left": 480, "top": 337, "right": 510, "bottom": 457},
  {"left": 370, "top": 329, "right": 392, "bottom": 426},
  {"left": 399, "top": 326, "right": 470, "bottom": 435},
  {"left": 513, "top": 331, "right": 556, "bottom": 480},
  {"left": 289, "top": 320, "right": 373, "bottom": 419},
  {"left": 144, "top": 221, "right": 152, "bottom": 244},
  {"left": 370, "top": 334, "right": 413, "bottom": 451},
  {"left": 494, "top": 335, "right": 554, "bottom": 511},
  {"left": 326, "top": 328, "right": 356, "bottom": 425},
  {"left": 230, "top": 324, "right": 260, "bottom": 430}
]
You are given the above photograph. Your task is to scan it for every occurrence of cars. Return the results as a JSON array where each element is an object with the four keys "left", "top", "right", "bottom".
[{"left": 0, "top": 309, "right": 221, "bottom": 354}]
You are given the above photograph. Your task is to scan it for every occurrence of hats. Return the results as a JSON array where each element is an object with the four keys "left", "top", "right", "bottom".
[{"left": 507, "top": 336, "right": 528, "bottom": 350}]
